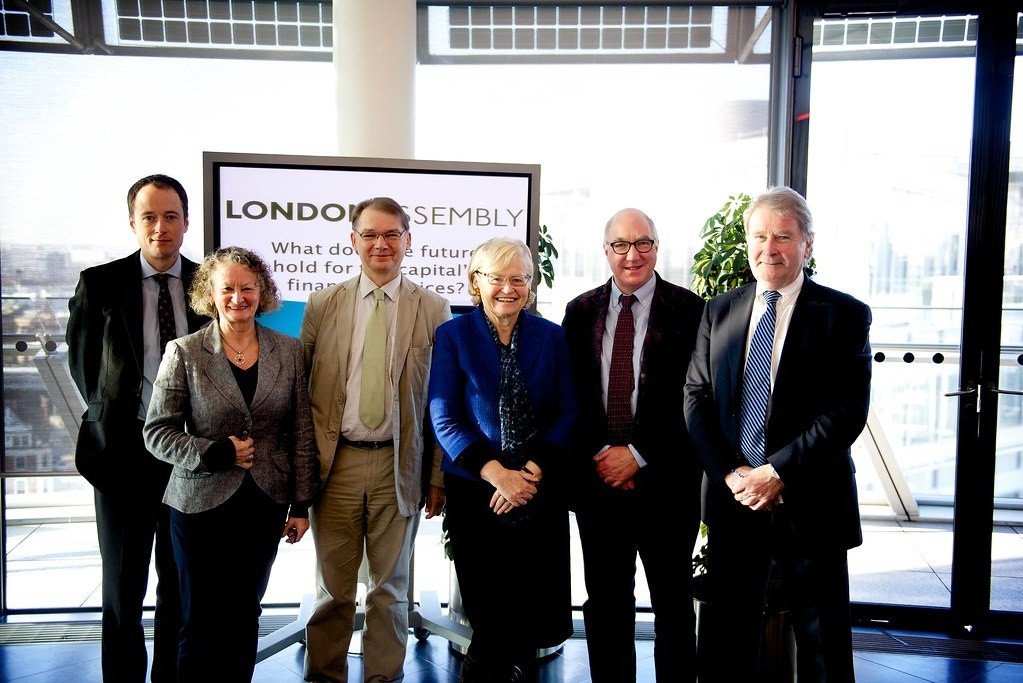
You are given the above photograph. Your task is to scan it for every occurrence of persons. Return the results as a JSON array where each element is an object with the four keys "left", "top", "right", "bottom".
[
  {"left": 560, "top": 208, "right": 707, "bottom": 683},
  {"left": 429, "top": 236, "right": 577, "bottom": 683},
  {"left": 65, "top": 174, "right": 219, "bottom": 683},
  {"left": 299, "top": 197, "right": 448, "bottom": 683},
  {"left": 683, "top": 185, "right": 873, "bottom": 683},
  {"left": 142, "top": 246, "right": 319, "bottom": 683}
]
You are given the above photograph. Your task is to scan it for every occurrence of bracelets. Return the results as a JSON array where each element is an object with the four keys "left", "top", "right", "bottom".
[{"left": 522, "top": 466, "right": 541, "bottom": 486}]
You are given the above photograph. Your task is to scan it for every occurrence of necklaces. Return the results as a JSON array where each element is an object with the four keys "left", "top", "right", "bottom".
[{"left": 219, "top": 326, "right": 257, "bottom": 366}]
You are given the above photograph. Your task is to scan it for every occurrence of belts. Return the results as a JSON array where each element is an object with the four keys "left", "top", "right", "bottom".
[{"left": 339, "top": 438, "right": 393, "bottom": 450}]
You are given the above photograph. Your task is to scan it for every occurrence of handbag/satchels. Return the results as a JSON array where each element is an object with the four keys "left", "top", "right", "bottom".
[{"left": 698, "top": 600, "right": 792, "bottom": 683}]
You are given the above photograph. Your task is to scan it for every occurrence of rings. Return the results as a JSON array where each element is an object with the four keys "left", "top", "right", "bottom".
[{"left": 248, "top": 455, "right": 254, "bottom": 461}]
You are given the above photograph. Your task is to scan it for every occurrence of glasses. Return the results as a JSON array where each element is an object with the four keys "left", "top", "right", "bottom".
[
  {"left": 472, "top": 270, "right": 530, "bottom": 287},
  {"left": 606, "top": 239, "right": 654, "bottom": 255},
  {"left": 353, "top": 228, "right": 407, "bottom": 243}
]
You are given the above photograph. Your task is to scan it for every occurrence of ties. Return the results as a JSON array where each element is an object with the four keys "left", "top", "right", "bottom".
[
  {"left": 606, "top": 294, "right": 638, "bottom": 448},
  {"left": 738, "top": 291, "right": 782, "bottom": 468},
  {"left": 151, "top": 273, "right": 177, "bottom": 361},
  {"left": 359, "top": 288, "right": 387, "bottom": 431}
]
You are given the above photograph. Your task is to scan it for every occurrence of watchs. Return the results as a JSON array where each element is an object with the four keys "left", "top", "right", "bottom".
[{"left": 769, "top": 463, "right": 784, "bottom": 487}]
